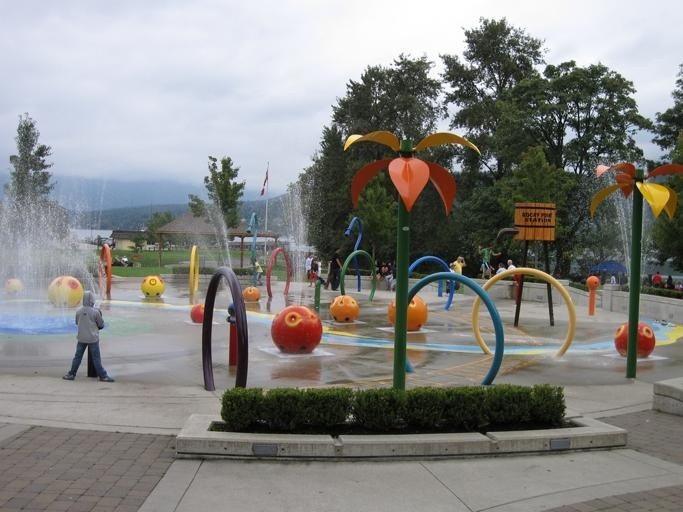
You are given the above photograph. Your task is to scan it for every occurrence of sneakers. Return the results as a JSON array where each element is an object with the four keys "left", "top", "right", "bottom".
[
  {"left": 62, "top": 375, "right": 74, "bottom": 380},
  {"left": 100, "top": 376, "right": 114, "bottom": 382}
]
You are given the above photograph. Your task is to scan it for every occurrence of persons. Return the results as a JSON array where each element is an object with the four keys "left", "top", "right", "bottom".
[
  {"left": 380, "top": 261, "right": 394, "bottom": 290},
  {"left": 496, "top": 260, "right": 518, "bottom": 274},
  {"left": 447, "top": 255, "right": 465, "bottom": 293},
  {"left": 62, "top": 291, "right": 115, "bottom": 382},
  {"left": 305, "top": 252, "right": 322, "bottom": 287},
  {"left": 330, "top": 253, "right": 343, "bottom": 291},
  {"left": 254, "top": 257, "right": 263, "bottom": 286}
]
